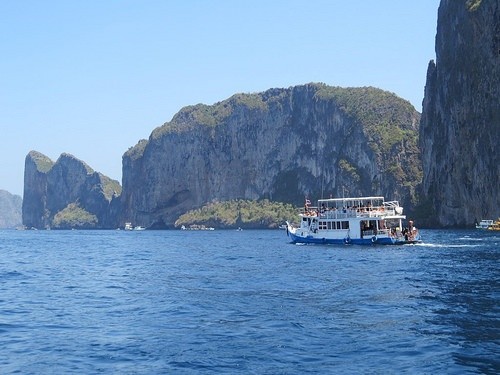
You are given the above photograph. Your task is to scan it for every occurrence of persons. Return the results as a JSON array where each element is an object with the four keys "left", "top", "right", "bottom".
[
  {"left": 402, "top": 220, "right": 418, "bottom": 241},
  {"left": 321, "top": 201, "right": 374, "bottom": 211}
]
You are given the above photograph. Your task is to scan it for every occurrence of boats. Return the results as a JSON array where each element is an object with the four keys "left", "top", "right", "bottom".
[{"left": 285, "top": 196, "right": 422, "bottom": 245}]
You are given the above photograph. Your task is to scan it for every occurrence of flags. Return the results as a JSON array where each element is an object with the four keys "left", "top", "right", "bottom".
[{"left": 305, "top": 196, "right": 311, "bottom": 204}]
